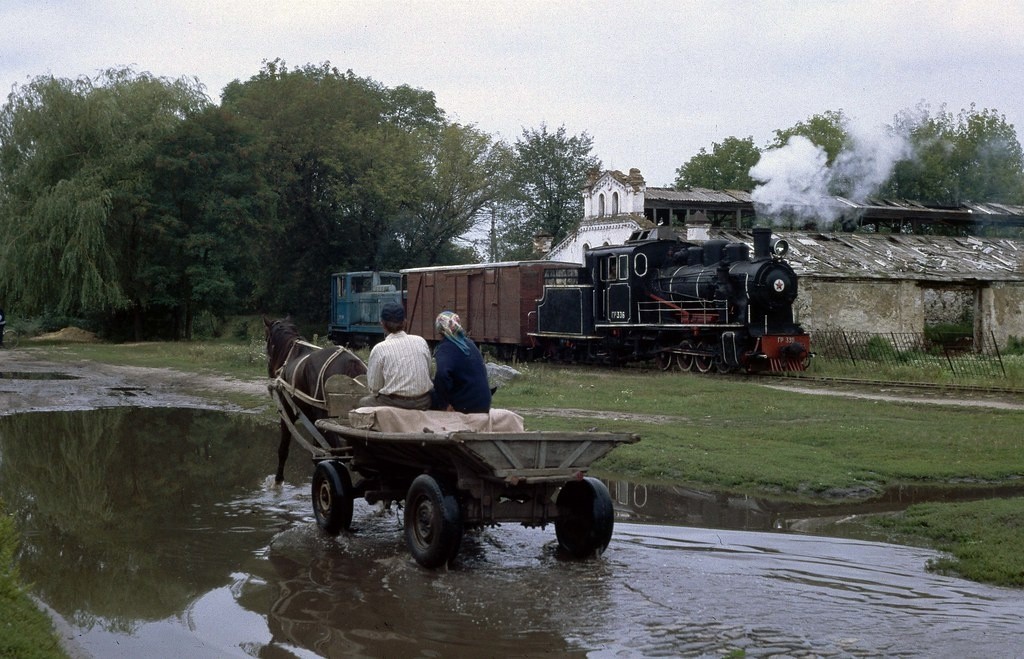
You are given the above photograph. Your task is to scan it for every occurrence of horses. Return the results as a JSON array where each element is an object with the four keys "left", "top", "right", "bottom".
[{"left": 263, "top": 313, "right": 368, "bottom": 485}]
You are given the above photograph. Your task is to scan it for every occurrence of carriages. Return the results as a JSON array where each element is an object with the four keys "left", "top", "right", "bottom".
[{"left": 262, "top": 317, "right": 643, "bottom": 573}]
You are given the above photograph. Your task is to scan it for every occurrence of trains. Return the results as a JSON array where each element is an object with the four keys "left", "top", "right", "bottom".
[{"left": 327, "top": 225, "right": 813, "bottom": 377}]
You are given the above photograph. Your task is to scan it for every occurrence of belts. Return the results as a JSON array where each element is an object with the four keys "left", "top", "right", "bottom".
[{"left": 389, "top": 388, "right": 434, "bottom": 401}]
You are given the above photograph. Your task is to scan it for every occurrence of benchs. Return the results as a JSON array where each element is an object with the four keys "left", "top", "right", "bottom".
[{"left": 348, "top": 408, "right": 525, "bottom": 437}]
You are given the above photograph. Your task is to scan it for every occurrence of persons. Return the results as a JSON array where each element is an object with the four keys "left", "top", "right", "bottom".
[
  {"left": 430, "top": 310, "right": 492, "bottom": 413},
  {"left": 358, "top": 304, "right": 435, "bottom": 411},
  {"left": 0, "top": 309, "right": 7, "bottom": 346}
]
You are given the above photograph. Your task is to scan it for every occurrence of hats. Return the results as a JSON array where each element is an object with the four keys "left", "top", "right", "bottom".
[{"left": 380, "top": 303, "right": 405, "bottom": 320}]
